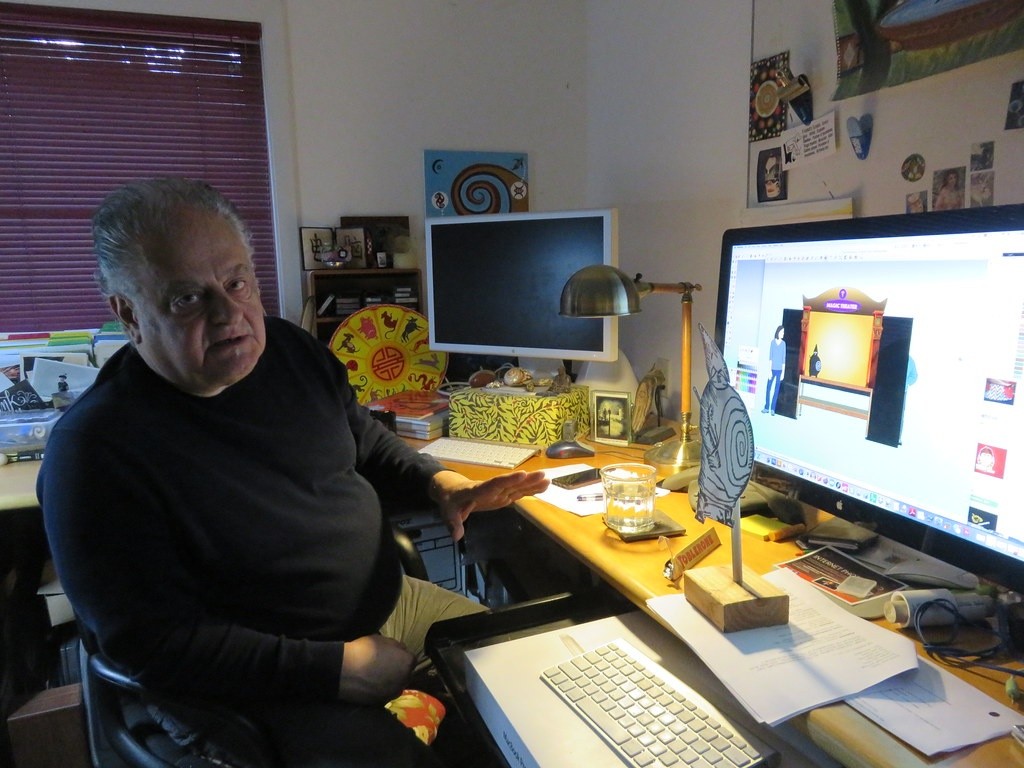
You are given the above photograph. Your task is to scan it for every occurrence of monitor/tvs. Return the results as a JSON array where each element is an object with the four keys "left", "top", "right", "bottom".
[
  {"left": 713, "top": 203, "right": 1024, "bottom": 600},
  {"left": 421, "top": 206, "right": 618, "bottom": 397}
]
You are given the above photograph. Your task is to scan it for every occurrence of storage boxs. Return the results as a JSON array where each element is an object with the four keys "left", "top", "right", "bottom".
[{"left": 449, "top": 377, "right": 590, "bottom": 448}]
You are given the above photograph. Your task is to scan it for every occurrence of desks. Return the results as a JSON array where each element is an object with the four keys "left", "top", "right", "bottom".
[{"left": 0, "top": 412, "right": 1024, "bottom": 768}]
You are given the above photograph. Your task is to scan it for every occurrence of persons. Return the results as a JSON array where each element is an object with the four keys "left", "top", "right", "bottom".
[
  {"left": 933, "top": 169, "right": 963, "bottom": 211},
  {"left": 36, "top": 179, "right": 550, "bottom": 768},
  {"left": 58, "top": 373, "right": 69, "bottom": 393}
]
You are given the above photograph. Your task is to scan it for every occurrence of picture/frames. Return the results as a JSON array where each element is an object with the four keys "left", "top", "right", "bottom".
[{"left": 590, "top": 390, "right": 632, "bottom": 447}]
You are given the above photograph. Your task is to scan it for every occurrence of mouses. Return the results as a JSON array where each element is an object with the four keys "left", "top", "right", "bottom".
[{"left": 546, "top": 439, "right": 595, "bottom": 460}]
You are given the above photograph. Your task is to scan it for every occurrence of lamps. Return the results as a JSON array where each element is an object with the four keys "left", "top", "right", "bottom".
[{"left": 557, "top": 262, "right": 703, "bottom": 479}]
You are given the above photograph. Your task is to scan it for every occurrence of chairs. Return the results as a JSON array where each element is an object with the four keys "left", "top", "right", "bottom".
[{"left": 35, "top": 460, "right": 446, "bottom": 768}]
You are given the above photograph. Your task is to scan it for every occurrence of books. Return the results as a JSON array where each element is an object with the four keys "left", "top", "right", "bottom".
[
  {"left": 362, "top": 390, "right": 450, "bottom": 420},
  {"left": 0, "top": 321, "right": 133, "bottom": 409},
  {"left": 318, "top": 284, "right": 419, "bottom": 317}
]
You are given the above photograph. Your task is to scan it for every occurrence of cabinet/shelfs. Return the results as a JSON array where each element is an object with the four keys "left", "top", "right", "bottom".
[{"left": 300, "top": 267, "right": 423, "bottom": 341}]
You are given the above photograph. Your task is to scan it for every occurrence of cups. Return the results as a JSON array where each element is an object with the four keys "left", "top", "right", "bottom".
[{"left": 599, "top": 463, "right": 656, "bottom": 533}]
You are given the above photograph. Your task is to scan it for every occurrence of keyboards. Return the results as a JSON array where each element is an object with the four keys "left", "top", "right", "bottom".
[
  {"left": 417, "top": 436, "right": 541, "bottom": 469},
  {"left": 540, "top": 638, "right": 781, "bottom": 768}
]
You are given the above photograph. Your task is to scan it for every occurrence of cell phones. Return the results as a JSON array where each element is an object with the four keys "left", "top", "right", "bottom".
[{"left": 552, "top": 468, "right": 602, "bottom": 490}]
